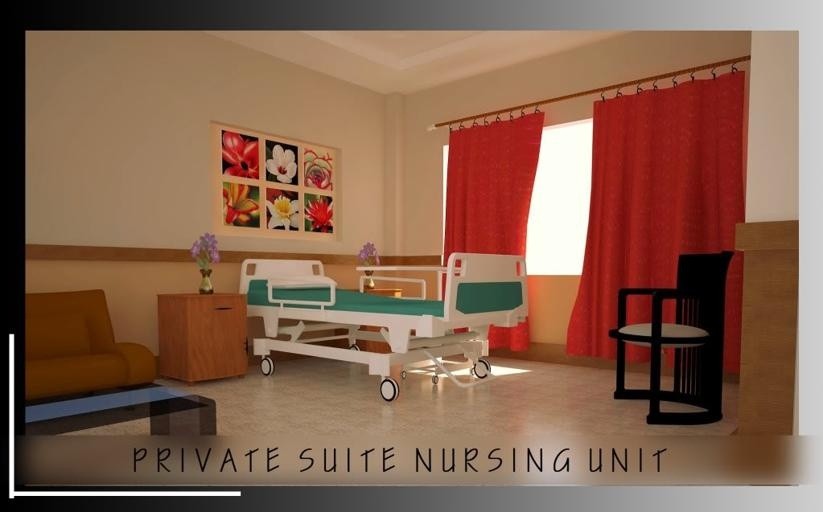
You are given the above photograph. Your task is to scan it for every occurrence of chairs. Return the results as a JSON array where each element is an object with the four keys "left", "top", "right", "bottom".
[{"left": 605, "top": 244, "right": 736, "bottom": 429}]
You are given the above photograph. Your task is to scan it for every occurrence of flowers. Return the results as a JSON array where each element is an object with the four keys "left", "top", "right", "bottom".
[
  {"left": 186, "top": 231, "right": 226, "bottom": 269},
  {"left": 356, "top": 242, "right": 382, "bottom": 272},
  {"left": 220, "top": 130, "right": 336, "bottom": 234}
]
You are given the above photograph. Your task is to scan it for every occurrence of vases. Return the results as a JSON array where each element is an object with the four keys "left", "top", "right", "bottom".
[
  {"left": 363, "top": 269, "right": 377, "bottom": 288},
  {"left": 196, "top": 267, "right": 215, "bottom": 293}
]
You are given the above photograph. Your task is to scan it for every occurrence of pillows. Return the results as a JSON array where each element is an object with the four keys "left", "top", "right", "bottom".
[{"left": 266, "top": 273, "right": 339, "bottom": 290}]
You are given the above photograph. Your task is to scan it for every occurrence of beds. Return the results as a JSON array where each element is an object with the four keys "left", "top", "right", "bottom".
[{"left": 234, "top": 252, "right": 530, "bottom": 410}]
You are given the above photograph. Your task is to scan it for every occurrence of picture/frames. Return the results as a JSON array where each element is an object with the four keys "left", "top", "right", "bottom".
[{"left": 206, "top": 121, "right": 345, "bottom": 246}]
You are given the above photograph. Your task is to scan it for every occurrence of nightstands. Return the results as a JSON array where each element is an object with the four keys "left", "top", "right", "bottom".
[
  {"left": 338, "top": 285, "right": 401, "bottom": 354},
  {"left": 155, "top": 288, "right": 252, "bottom": 390}
]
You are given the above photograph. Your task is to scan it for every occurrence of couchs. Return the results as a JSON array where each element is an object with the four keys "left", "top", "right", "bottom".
[{"left": 25, "top": 287, "right": 158, "bottom": 403}]
